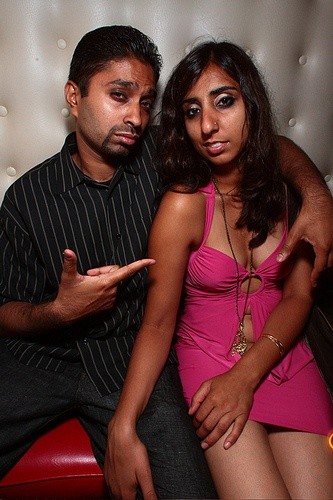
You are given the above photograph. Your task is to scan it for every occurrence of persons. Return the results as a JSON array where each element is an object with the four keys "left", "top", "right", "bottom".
[
  {"left": 103, "top": 41, "right": 333, "bottom": 500},
  {"left": 0, "top": 25, "right": 333, "bottom": 500}
]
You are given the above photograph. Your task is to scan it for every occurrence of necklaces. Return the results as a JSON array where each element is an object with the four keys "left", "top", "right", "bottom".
[{"left": 210, "top": 172, "right": 255, "bottom": 356}]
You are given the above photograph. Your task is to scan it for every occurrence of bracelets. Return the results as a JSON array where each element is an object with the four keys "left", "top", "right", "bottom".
[{"left": 259, "top": 333, "right": 287, "bottom": 359}]
